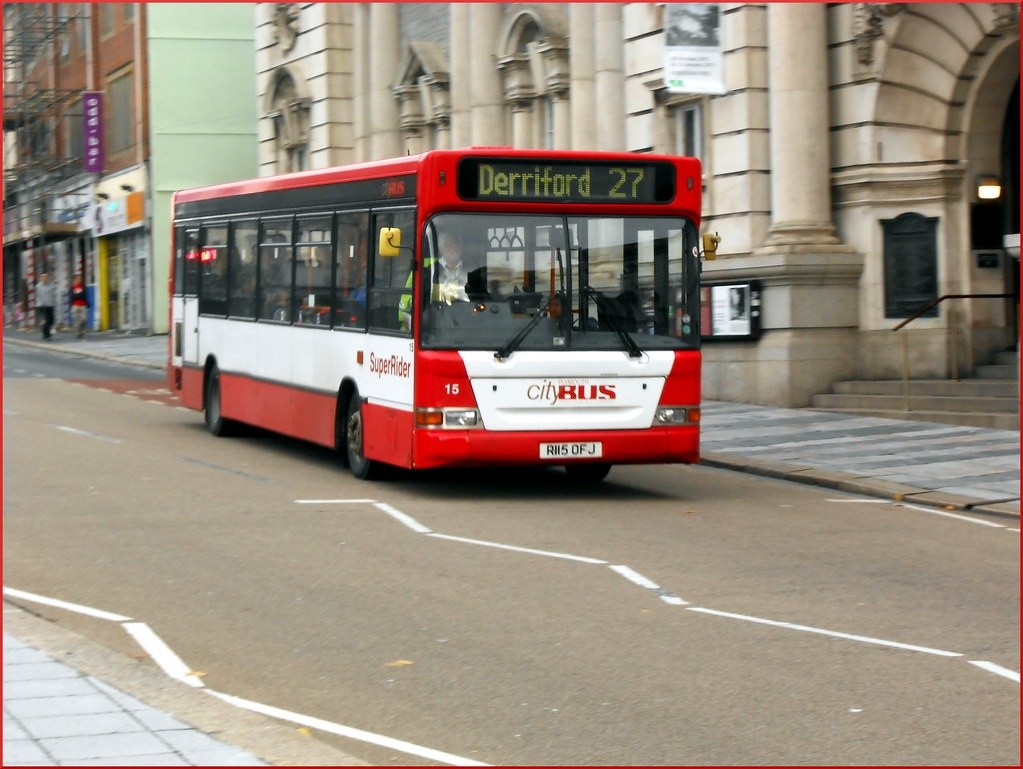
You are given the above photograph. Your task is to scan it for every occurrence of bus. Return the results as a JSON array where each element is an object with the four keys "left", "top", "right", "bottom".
[{"left": 161, "top": 146, "right": 721, "bottom": 491}]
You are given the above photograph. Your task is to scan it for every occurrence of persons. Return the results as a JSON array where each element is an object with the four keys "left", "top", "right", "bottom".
[
  {"left": 401, "top": 230, "right": 476, "bottom": 331},
  {"left": 201, "top": 235, "right": 341, "bottom": 320},
  {"left": 32, "top": 274, "right": 58, "bottom": 343},
  {"left": 68, "top": 276, "right": 92, "bottom": 340}
]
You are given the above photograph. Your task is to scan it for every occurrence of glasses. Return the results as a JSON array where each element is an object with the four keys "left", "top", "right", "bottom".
[{"left": 444, "top": 241, "right": 461, "bottom": 248}]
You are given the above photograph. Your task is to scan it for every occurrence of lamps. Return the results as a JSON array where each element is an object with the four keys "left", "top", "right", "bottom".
[
  {"left": 96, "top": 194, "right": 109, "bottom": 200},
  {"left": 1004, "top": 234, "right": 1021, "bottom": 258},
  {"left": 974, "top": 173, "right": 1001, "bottom": 199},
  {"left": 121, "top": 185, "right": 132, "bottom": 191}
]
